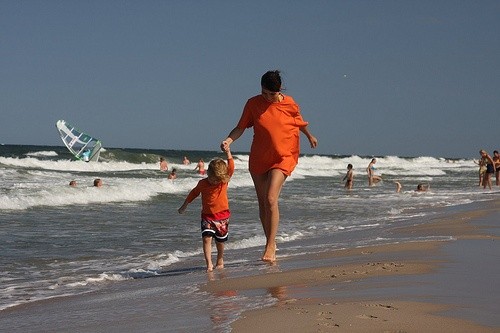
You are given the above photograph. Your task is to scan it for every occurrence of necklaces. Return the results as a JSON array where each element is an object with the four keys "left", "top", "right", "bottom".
[{"left": 278, "top": 95, "right": 282, "bottom": 102}]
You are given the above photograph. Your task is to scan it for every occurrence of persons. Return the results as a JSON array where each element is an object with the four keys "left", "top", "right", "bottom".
[
  {"left": 178, "top": 143, "right": 235, "bottom": 273},
  {"left": 474, "top": 150, "right": 500, "bottom": 190},
  {"left": 343, "top": 158, "right": 426, "bottom": 193},
  {"left": 160, "top": 156, "right": 206, "bottom": 179},
  {"left": 69, "top": 179, "right": 103, "bottom": 187},
  {"left": 220, "top": 70, "right": 318, "bottom": 266}
]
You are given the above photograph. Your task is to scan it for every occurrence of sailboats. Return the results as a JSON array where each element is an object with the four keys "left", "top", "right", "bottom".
[{"left": 56, "top": 120, "right": 101, "bottom": 163}]
